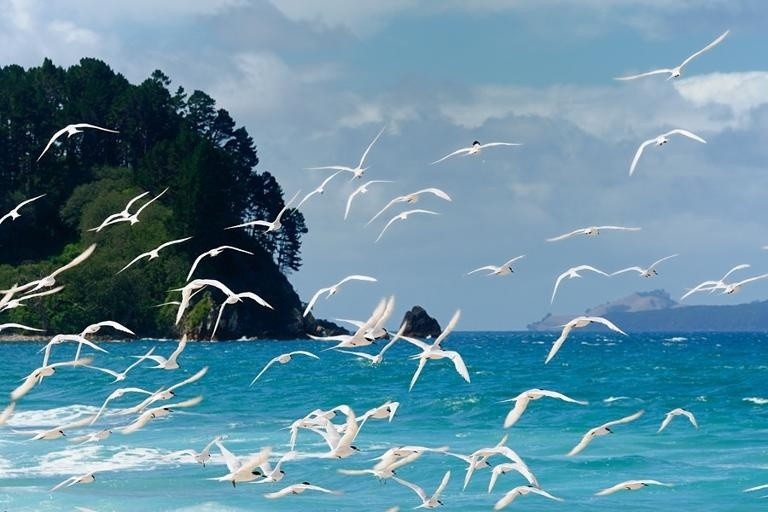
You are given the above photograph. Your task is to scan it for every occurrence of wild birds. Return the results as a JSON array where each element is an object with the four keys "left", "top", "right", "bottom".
[
  {"left": 0, "top": 122, "right": 768, "bottom": 512},
  {"left": 614, "top": 26, "right": 732, "bottom": 82}
]
